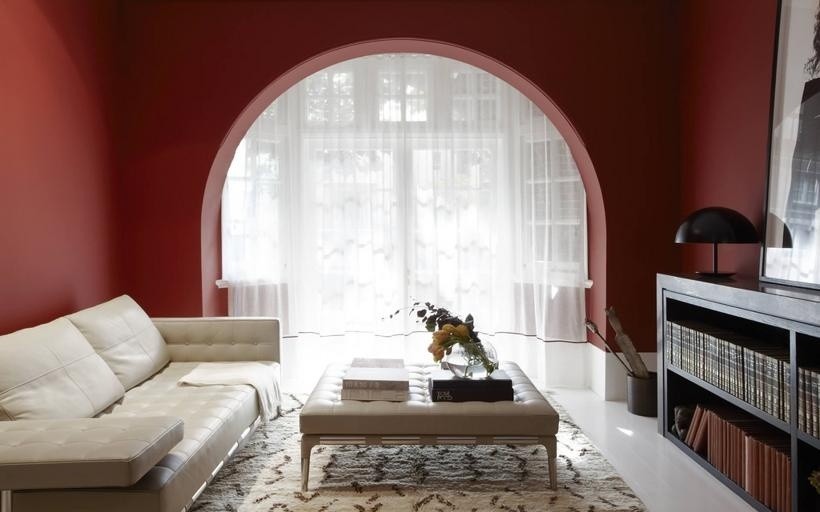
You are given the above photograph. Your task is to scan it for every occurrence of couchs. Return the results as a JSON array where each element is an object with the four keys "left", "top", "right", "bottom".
[{"left": 0, "top": 309, "right": 279, "bottom": 512}]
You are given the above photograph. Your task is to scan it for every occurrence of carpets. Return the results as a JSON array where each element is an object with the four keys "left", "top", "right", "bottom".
[{"left": 186, "top": 389, "right": 648, "bottom": 512}]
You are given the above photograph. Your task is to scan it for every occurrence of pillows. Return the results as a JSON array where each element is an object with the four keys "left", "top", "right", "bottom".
[
  {"left": 65, "top": 294, "right": 172, "bottom": 390},
  {"left": 0, "top": 316, "right": 125, "bottom": 418}
]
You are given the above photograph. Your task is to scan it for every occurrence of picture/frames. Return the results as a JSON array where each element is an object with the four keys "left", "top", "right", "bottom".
[{"left": 759, "top": 0, "right": 820, "bottom": 290}]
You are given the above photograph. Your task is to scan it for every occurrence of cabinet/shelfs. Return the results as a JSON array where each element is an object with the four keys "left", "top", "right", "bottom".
[{"left": 654, "top": 272, "right": 820, "bottom": 512}]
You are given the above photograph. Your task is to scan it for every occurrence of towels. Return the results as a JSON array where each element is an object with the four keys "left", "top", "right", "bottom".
[{"left": 177, "top": 362, "right": 282, "bottom": 423}]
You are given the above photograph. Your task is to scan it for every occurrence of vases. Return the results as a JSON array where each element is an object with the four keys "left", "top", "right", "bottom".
[{"left": 443, "top": 340, "right": 497, "bottom": 380}]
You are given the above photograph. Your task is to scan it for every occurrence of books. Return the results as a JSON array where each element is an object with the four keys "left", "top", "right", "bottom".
[
  {"left": 665, "top": 317, "right": 820, "bottom": 512},
  {"left": 340, "top": 352, "right": 412, "bottom": 402}
]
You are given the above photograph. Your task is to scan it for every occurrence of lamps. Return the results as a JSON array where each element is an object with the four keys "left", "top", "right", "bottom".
[{"left": 674, "top": 207, "right": 762, "bottom": 275}]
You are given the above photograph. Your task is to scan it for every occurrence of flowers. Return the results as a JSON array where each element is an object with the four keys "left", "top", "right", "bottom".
[{"left": 383, "top": 297, "right": 499, "bottom": 373}]
